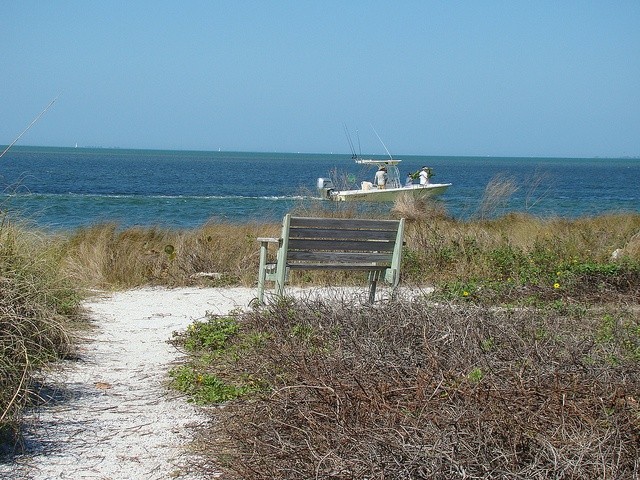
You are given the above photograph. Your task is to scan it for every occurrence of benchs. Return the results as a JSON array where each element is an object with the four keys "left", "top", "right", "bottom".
[{"left": 257, "top": 214, "right": 405, "bottom": 305}]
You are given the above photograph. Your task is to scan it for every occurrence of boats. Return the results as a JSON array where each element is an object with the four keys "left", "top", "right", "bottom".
[
  {"left": 356, "top": 158, "right": 403, "bottom": 165},
  {"left": 316, "top": 165, "right": 452, "bottom": 203}
]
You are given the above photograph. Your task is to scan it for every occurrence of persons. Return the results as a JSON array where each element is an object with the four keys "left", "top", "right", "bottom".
[
  {"left": 416, "top": 165, "right": 430, "bottom": 188},
  {"left": 373, "top": 166, "right": 387, "bottom": 191}
]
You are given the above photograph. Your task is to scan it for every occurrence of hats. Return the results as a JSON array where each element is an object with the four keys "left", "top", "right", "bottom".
[
  {"left": 378, "top": 165, "right": 385, "bottom": 170},
  {"left": 422, "top": 167, "right": 428, "bottom": 169}
]
